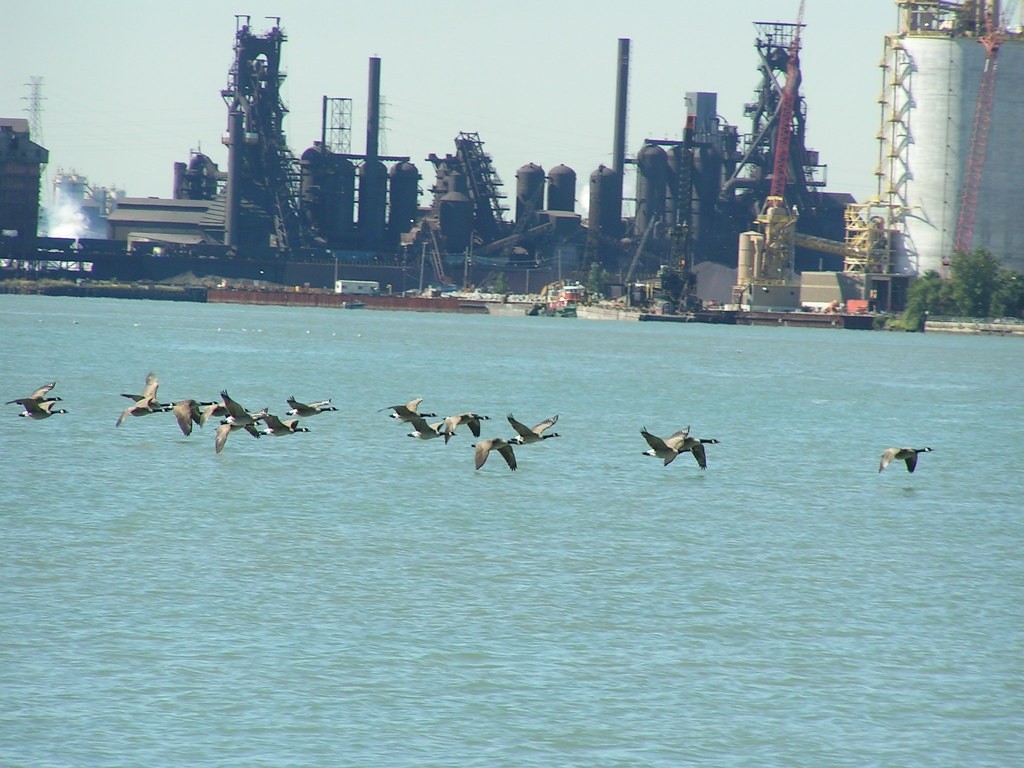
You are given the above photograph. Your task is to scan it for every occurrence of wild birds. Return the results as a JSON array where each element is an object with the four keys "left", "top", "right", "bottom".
[
  {"left": 283, "top": 396, "right": 338, "bottom": 418},
  {"left": 4, "top": 381, "right": 68, "bottom": 420},
  {"left": 877, "top": 447, "right": 933, "bottom": 473},
  {"left": 470, "top": 413, "right": 561, "bottom": 471},
  {"left": 377, "top": 397, "right": 490, "bottom": 446},
  {"left": 641, "top": 427, "right": 720, "bottom": 469},
  {"left": 115, "top": 373, "right": 312, "bottom": 454}
]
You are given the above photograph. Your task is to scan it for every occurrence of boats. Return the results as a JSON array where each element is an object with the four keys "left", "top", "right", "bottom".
[
  {"left": 528, "top": 282, "right": 584, "bottom": 318},
  {"left": 340, "top": 299, "right": 366, "bottom": 309},
  {"left": 626, "top": 191, "right": 905, "bottom": 330}
]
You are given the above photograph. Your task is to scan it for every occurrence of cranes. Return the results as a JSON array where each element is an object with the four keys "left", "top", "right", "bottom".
[
  {"left": 950, "top": 0, "right": 1011, "bottom": 281},
  {"left": 769, "top": 0, "right": 809, "bottom": 205}
]
